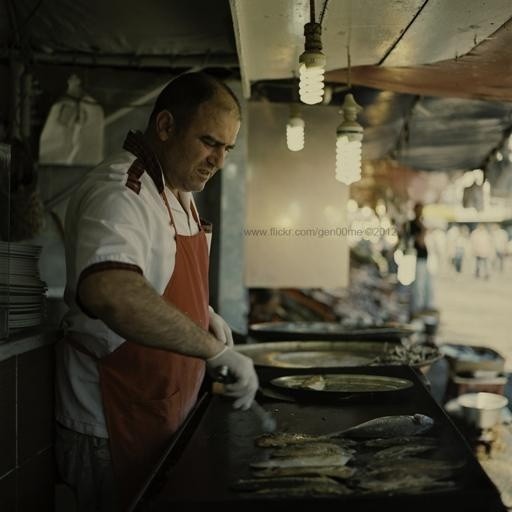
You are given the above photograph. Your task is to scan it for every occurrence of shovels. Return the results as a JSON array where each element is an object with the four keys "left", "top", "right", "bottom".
[{"left": 215, "top": 364, "right": 296, "bottom": 403}]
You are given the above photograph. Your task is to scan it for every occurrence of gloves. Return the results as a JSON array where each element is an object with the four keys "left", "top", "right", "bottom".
[
  {"left": 206, "top": 346, "right": 259, "bottom": 410},
  {"left": 208, "top": 304, "right": 234, "bottom": 345}
]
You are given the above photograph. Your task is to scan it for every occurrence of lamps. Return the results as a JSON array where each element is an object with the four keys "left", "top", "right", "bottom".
[
  {"left": 335, "top": 46, "right": 364, "bottom": 184},
  {"left": 299, "top": 0, "right": 327, "bottom": 105},
  {"left": 286, "top": 70, "right": 305, "bottom": 151}
]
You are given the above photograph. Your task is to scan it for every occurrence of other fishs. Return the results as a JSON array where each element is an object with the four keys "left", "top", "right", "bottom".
[{"left": 230, "top": 411, "right": 472, "bottom": 502}]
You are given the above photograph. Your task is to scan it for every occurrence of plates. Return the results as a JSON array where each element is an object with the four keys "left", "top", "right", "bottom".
[
  {"left": 1, "top": 242, "right": 46, "bottom": 333},
  {"left": 269, "top": 373, "right": 414, "bottom": 399}
]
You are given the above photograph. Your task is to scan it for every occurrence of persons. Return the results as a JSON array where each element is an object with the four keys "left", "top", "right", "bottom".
[
  {"left": 400, "top": 201, "right": 509, "bottom": 317},
  {"left": 54, "top": 71, "right": 260, "bottom": 512}
]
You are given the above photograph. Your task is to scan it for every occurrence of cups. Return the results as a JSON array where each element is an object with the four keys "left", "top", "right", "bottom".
[{"left": 459, "top": 392, "right": 509, "bottom": 442}]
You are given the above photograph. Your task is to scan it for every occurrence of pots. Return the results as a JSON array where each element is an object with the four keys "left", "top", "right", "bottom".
[{"left": 231, "top": 320, "right": 443, "bottom": 375}]
